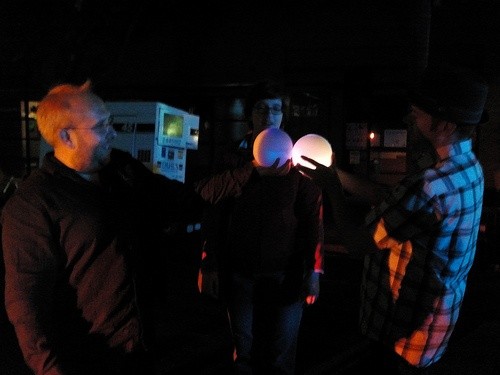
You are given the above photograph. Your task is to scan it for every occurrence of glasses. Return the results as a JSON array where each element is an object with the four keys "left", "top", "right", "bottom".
[
  {"left": 252, "top": 105, "right": 282, "bottom": 115},
  {"left": 53, "top": 116, "right": 113, "bottom": 136}
]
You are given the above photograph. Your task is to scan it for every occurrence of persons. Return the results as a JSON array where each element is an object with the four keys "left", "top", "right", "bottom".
[
  {"left": 297, "top": 62, "right": 485, "bottom": 375},
  {"left": 196, "top": 80, "right": 328, "bottom": 375},
  {"left": 0, "top": 78, "right": 290, "bottom": 375}
]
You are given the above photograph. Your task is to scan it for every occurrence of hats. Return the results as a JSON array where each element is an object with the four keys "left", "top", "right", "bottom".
[{"left": 408, "top": 69, "right": 490, "bottom": 126}]
